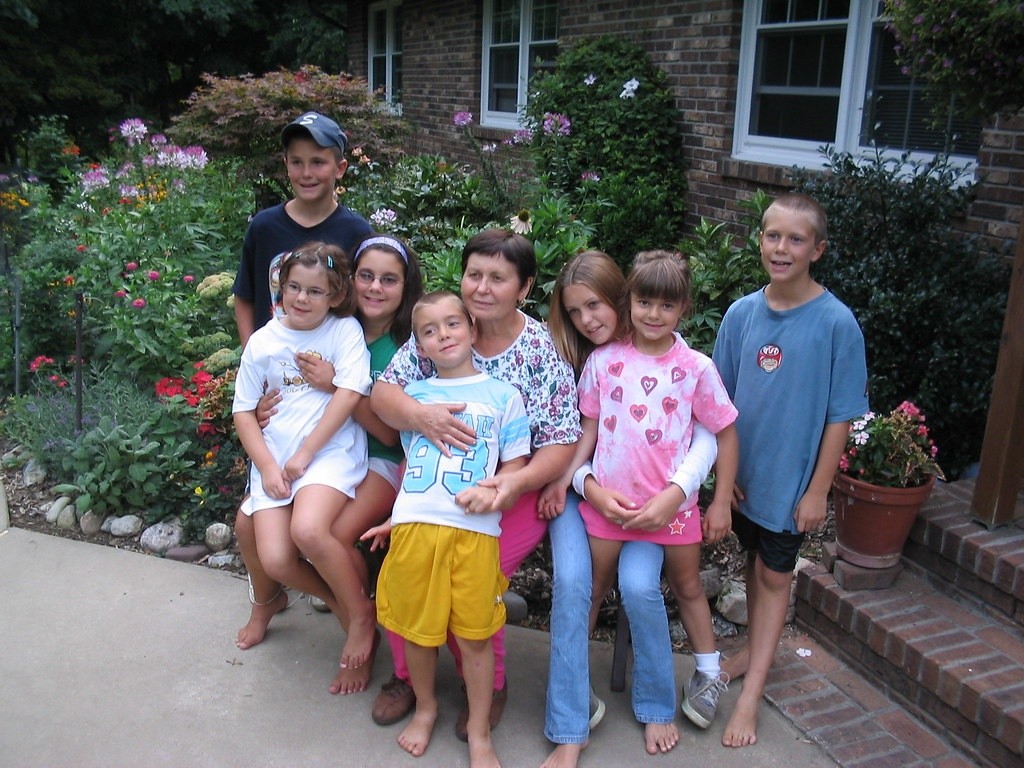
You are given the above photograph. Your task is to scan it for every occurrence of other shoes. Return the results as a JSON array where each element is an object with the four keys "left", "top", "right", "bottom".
[{"left": 308, "top": 594, "right": 331, "bottom": 612}]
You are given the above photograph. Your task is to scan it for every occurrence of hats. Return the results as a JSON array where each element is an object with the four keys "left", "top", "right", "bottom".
[{"left": 278, "top": 111, "right": 348, "bottom": 159}]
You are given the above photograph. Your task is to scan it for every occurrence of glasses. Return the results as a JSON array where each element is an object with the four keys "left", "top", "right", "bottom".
[
  {"left": 281, "top": 280, "right": 331, "bottom": 300},
  {"left": 353, "top": 269, "right": 405, "bottom": 288}
]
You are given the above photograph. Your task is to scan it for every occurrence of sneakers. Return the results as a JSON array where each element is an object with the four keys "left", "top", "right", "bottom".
[
  {"left": 588, "top": 682, "right": 605, "bottom": 730},
  {"left": 455, "top": 676, "right": 508, "bottom": 742},
  {"left": 371, "top": 671, "right": 417, "bottom": 726},
  {"left": 680, "top": 669, "right": 730, "bottom": 729}
]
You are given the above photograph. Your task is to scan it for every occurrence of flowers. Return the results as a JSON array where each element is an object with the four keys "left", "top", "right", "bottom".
[{"left": 838, "top": 401, "right": 946, "bottom": 488}]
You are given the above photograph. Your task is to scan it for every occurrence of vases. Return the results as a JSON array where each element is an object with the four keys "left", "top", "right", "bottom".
[{"left": 832, "top": 472, "right": 936, "bottom": 568}]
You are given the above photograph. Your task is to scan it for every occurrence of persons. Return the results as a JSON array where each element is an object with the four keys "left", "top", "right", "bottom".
[
  {"left": 229, "top": 111, "right": 738, "bottom": 767},
  {"left": 711, "top": 195, "right": 869, "bottom": 748}
]
